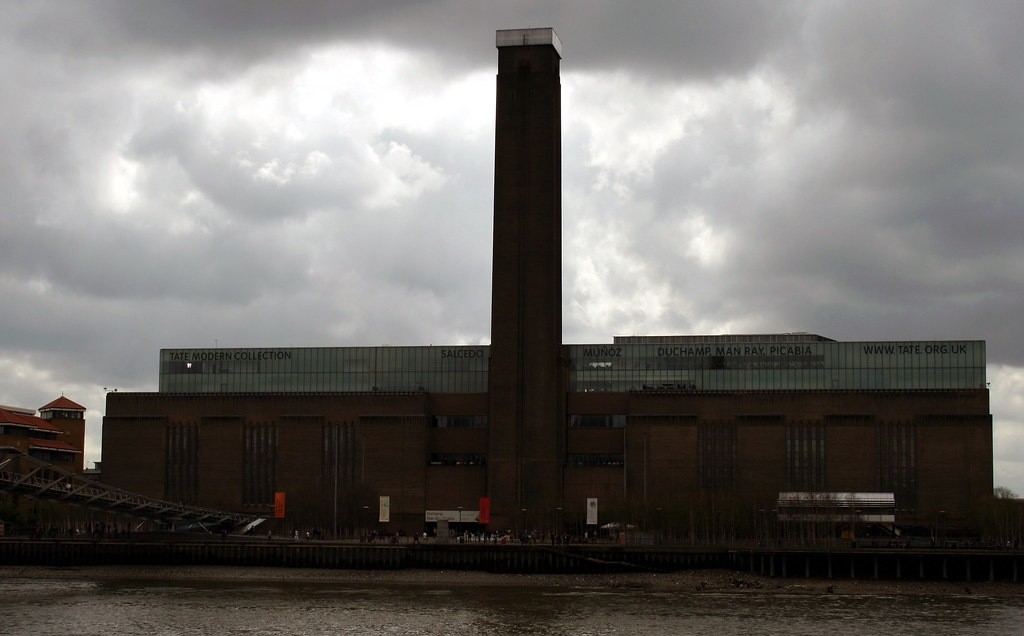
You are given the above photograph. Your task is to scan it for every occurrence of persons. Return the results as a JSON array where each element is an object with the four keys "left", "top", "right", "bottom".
[
  {"left": 291, "top": 528, "right": 311, "bottom": 542},
  {"left": 394, "top": 531, "right": 400, "bottom": 544},
  {"left": 268, "top": 529, "right": 272, "bottom": 541},
  {"left": 360, "top": 528, "right": 385, "bottom": 544},
  {"left": 423, "top": 532, "right": 428, "bottom": 544},
  {"left": 584, "top": 529, "right": 597, "bottom": 544},
  {"left": 522, "top": 529, "right": 561, "bottom": 545},
  {"left": 251, "top": 525, "right": 255, "bottom": 535},
  {"left": 413, "top": 532, "right": 420, "bottom": 544},
  {"left": 449, "top": 528, "right": 514, "bottom": 545}
]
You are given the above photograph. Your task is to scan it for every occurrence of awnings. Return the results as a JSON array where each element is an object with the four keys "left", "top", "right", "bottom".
[{"left": 601, "top": 523, "right": 637, "bottom": 529}]
